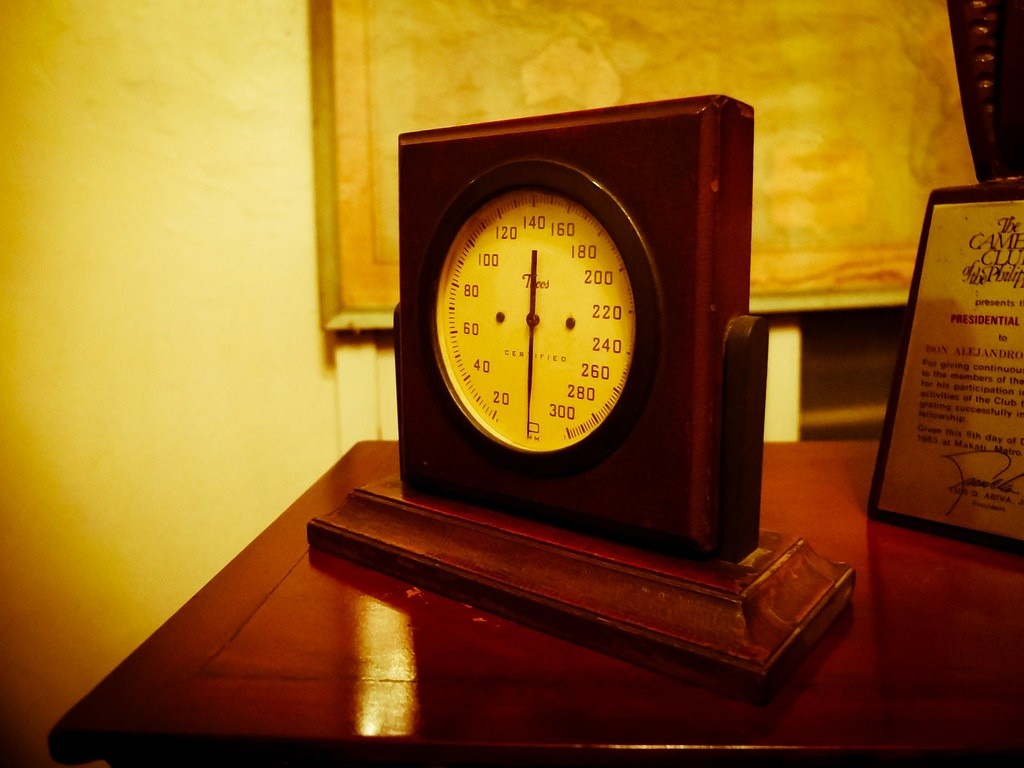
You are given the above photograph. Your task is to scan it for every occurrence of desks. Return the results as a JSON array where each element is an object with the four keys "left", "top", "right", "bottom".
[{"left": 41, "top": 436, "right": 1024, "bottom": 766}]
